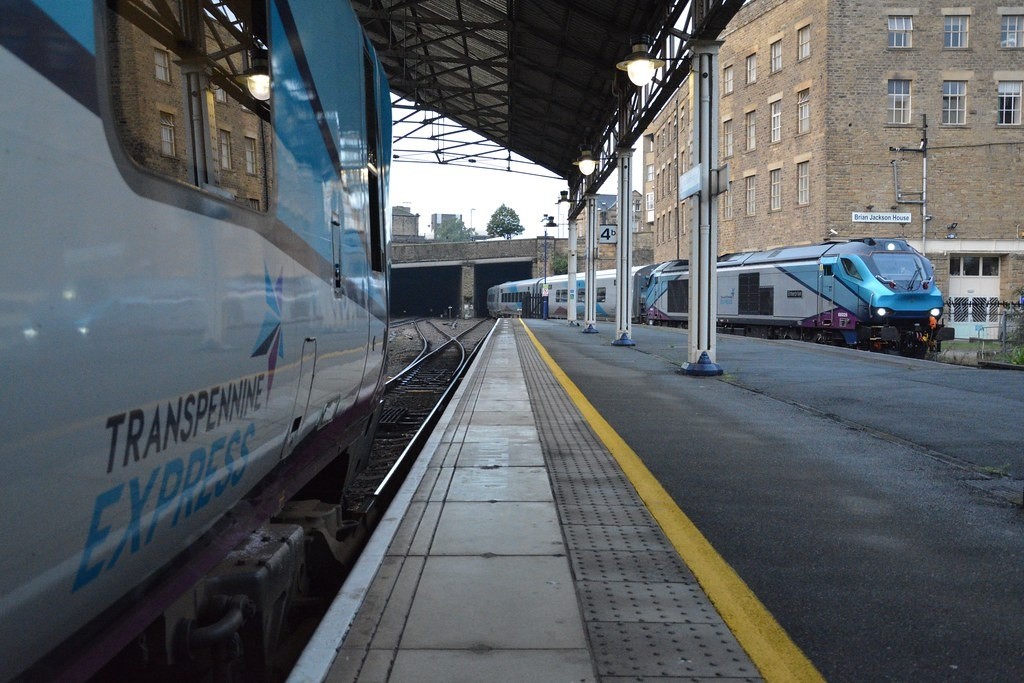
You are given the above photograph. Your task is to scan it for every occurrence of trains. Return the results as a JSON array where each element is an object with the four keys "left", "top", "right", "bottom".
[
  {"left": 0, "top": 0, "right": 392, "bottom": 683},
  {"left": 487, "top": 236, "right": 944, "bottom": 360}
]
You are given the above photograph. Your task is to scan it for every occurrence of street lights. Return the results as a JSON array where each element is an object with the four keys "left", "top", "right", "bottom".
[
  {"left": 470, "top": 208, "right": 476, "bottom": 234},
  {"left": 540, "top": 214, "right": 550, "bottom": 320}
]
